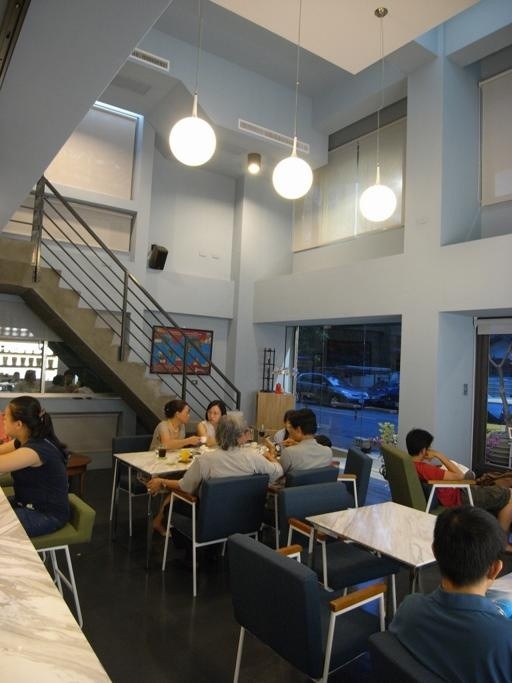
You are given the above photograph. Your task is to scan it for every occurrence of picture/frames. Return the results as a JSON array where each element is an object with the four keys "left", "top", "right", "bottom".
[{"left": 150, "top": 325, "right": 213, "bottom": 375}]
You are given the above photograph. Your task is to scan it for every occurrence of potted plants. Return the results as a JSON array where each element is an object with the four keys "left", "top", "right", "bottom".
[{"left": 376, "top": 420, "right": 399, "bottom": 480}]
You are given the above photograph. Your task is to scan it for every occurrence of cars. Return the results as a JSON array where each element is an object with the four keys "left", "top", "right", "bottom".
[{"left": 298, "top": 364, "right": 400, "bottom": 410}]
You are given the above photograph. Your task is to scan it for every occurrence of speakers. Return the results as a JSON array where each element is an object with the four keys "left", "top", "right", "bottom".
[{"left": 148, "top": 245, "right": 168, "bottom": 270}]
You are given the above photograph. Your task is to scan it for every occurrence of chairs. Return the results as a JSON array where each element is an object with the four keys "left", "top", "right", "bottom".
[{"left": 0, "top": 470, "right": 96, "bottom": 628}]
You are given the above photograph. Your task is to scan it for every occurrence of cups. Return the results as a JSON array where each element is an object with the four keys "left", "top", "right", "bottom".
[
  {"left": 3, "top": 356, "right": 54, "bottom": 369},
  {"left": 181, "top": 437, "right": 211, "bottom": 462},
  {"left": 245, "top": 441, "right": 269, "bottom": 457},
  {"left": 158, "top": 443, "right": 166, "bottom": 458}
]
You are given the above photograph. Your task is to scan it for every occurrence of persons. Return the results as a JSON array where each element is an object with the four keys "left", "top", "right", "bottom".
[
  {"left": 406, "top": 429, "right": 511, "bottom": 509},
  {"left": 2, "top": 370, "right": 80, "bottom": 394},
  {"left": 496, "top": 504, "right": 511, "bottom": 579},
  {"left": 388, "top": 506, "right": 511, "bottom": 683},
  {"left": 147, "top": 400, "right": 332, "bottom": 571},
  {"left": 1, "top": 396, "right": 71, "bottom": 537}
]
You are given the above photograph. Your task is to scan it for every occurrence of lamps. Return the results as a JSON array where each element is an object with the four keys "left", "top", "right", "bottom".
[
  {"left": 271, "top": 0, "right": 313, "bottom": 199},
  {"left": 248, "top": 152, "right": 261, "bottom": 174},
  {"left": 169, "top": 0, "right": 216, "bottom": 167},
  {"left": 359, "top": 8, "right": 397, "bottom": 221}
]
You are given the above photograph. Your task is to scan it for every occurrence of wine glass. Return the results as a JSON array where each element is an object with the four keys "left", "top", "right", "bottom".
[{"left": 259, "top": 423, "right": 265, "bottom": 447}]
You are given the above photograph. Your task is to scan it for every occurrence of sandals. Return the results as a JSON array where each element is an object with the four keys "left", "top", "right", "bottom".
[{"left": 151, "top": 522, "right": 172, "bottom": 538}]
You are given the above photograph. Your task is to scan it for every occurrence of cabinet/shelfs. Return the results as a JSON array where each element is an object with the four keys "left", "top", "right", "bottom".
[
  {"left": 257, "top": 389, "right": 296, "bottom": 444},
  {"left": 0, "top": 338, "right": 59, "bottom": 383}
]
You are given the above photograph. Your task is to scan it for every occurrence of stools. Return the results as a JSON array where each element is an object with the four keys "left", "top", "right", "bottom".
[{"left": 64, "top": 449, "right": 93, "bottom": 497}]
